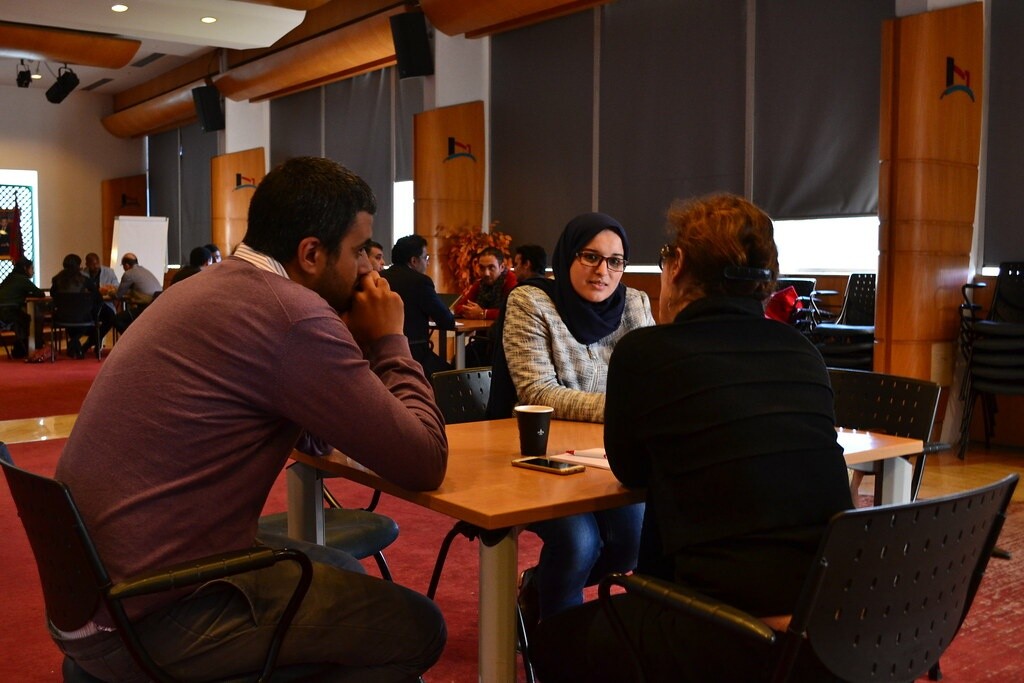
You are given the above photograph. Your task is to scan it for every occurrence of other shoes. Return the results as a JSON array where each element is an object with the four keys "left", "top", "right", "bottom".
[
  {"left": 66, "top": 340, "right": 88, "bottom": 359},
  {"left": 94, "top": 339, "right": 102, "bottom": 353},
  {"left": 515, "top": 567, "right": 541, "bottom": 653}
]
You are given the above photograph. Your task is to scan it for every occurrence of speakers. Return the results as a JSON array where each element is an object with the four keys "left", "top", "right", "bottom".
[
  {"left": 16, "top": 70, "right": 31, "bottom": 88},
  {"left": 389, "top": 8, "right": 435, "bottom": 79},
  {"left": 192, "top": 86, "right": 225, "bottom": 131},
  {"left": 45, "top": 71, "right": 80, "bottom": 104}
]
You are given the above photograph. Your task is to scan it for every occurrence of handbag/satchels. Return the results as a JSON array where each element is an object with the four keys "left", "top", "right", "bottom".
[{"left": 766, "top": 286, "right": 803, "bottom": 324}]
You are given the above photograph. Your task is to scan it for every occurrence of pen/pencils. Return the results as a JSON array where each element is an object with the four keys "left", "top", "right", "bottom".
[{"left": 566, "top": 450, "right": 606, "bottom": 458}]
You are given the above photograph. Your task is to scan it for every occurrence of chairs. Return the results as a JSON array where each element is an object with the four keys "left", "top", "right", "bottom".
[{"left": 0, "top": 260, "right": 1024, "bottom": 683}]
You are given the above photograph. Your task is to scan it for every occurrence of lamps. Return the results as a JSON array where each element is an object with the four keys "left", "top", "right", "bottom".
[
  {"left": 15, "top": 58, "right": 32, "bottom": 88},
  {"left": 45, "top": 65, "right": 80, "bottom": 105}
]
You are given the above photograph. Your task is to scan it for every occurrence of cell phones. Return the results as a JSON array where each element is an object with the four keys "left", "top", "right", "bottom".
[{"left": 512, "top": 456, "right": 586, "bottom": 475}]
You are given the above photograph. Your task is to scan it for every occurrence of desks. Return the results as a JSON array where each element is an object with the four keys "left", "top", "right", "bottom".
[
  {"left": 428, "top": 318, "right": 496, "bottom": 371},
  {"left": 24, "top": 295, "right": 124, "bottom": 360},
  {"left": 283, "top": 418, "right": 924, "bottom": 683}
]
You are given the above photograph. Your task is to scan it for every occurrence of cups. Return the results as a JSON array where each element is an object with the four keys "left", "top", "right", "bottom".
[{"left": 515, "top": 405, "right": 555, "bottom": 456}]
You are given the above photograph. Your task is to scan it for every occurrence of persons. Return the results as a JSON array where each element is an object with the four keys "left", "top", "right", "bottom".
[
  {"left": 55, "top": 156, "right": 450, "bottom": 683},
  {"left": 529, "top": 192, "right": 850, "bottom": 683},
  {"left": 495, "top": 212, "right": 644, "bottom": 635},
  {"left": 0, "top": 236, "right": 546, "bottom": 426}
]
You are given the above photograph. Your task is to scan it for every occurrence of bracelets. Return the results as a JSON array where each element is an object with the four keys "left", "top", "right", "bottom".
[
  {"left": 480, "top": 308, "right": 484, "bottom": 320},
  {"left": 484, "top": 308, "right": 488, "bottom": 320}
]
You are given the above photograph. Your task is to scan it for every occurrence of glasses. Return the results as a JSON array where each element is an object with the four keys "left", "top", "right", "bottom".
[
  {"left": 575, "top": 252, "right": 628, "bottom": 272},
  {"left": 421, "top": 255, "right": 430, "bottom": 261},
  {"left": 655, "top": 248, "right": 675, "bottom": 270}
]
